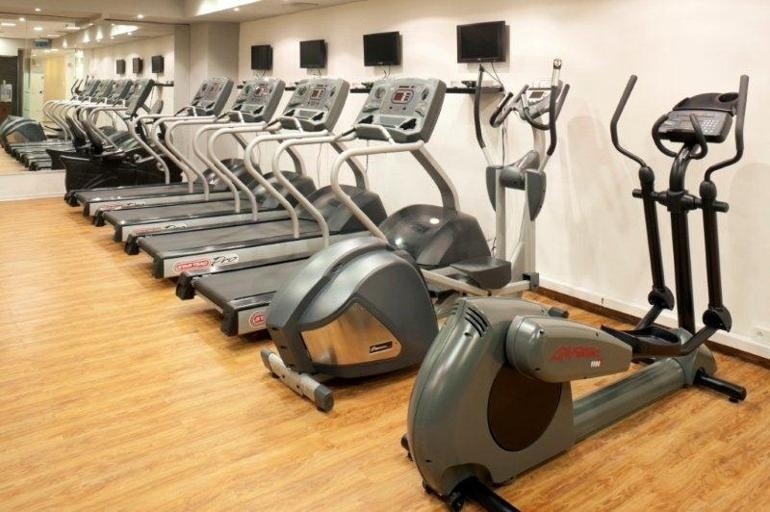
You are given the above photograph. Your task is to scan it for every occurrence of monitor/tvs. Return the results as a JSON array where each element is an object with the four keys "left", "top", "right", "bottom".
[
  {"left": 151, "top": 56, "right": 163, "bottom": 73},
  {"left": 116, "top": 60, "right": 125, "bottom": 74},
  {"left": 300, "top": 40, "right": 324, "bottom": 68},
  {"left": 251, "top": 45, "right": 272, "bottom": 70},
  {"left": 133, "top": 58, "right": 142, "bottom": 73},
  {"left": 457, "top": 21, "right": 506, "bottom": 62},
  {"left": 363, "top": 31, "right": 401, "bottom": 66}
]
